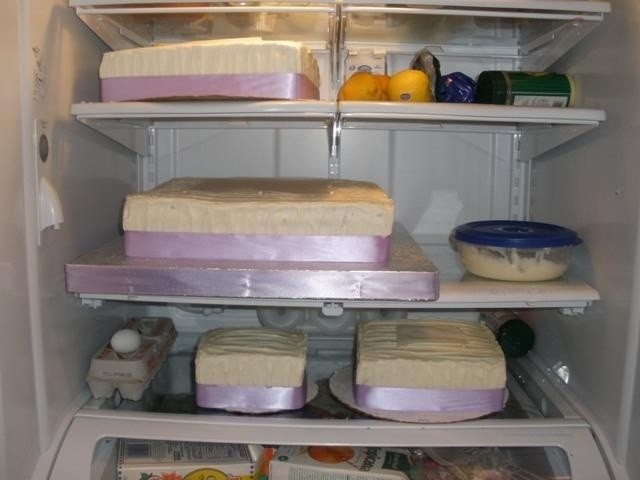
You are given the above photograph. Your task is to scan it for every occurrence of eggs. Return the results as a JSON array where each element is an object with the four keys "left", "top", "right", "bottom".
[{"left": 111, "top": 327, "right": 142, "bottom": 353}]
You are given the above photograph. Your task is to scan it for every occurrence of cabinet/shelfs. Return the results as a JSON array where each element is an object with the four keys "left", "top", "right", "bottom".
[{"left": 0, "top": 4, "right": 640, "bottom": 479}]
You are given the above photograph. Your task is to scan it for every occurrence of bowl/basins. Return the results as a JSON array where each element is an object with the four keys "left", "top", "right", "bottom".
[{"left": 455, "top": 220, "right": 584, "bottom": 281}]
